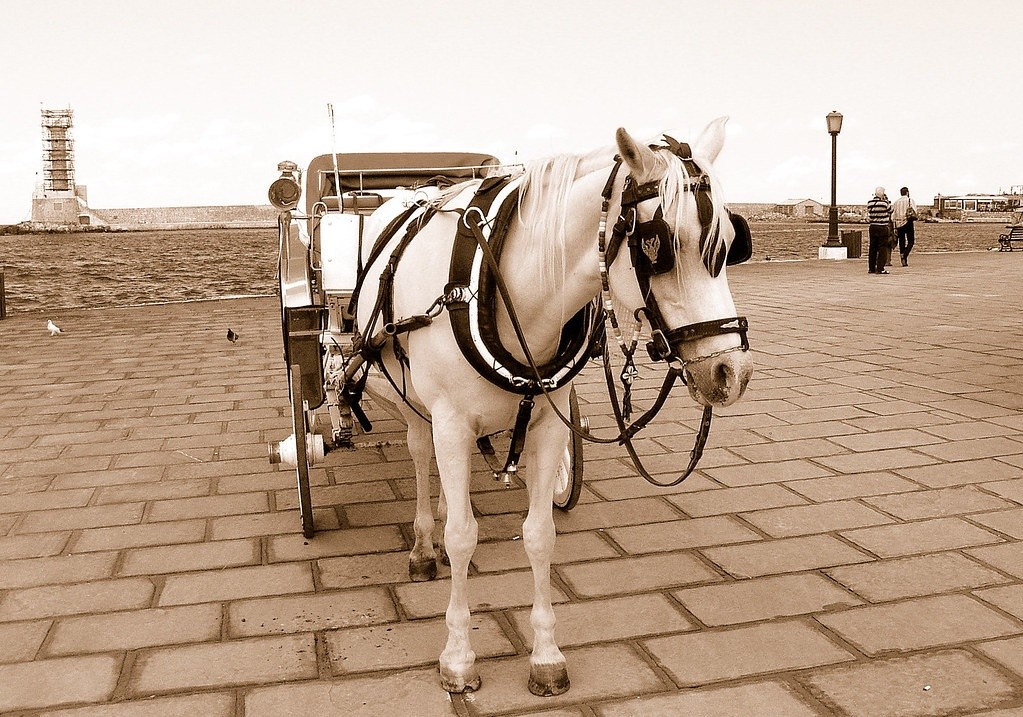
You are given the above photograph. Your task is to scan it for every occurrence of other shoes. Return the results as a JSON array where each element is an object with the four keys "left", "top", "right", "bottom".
[
  {"left": 885, "top": 262, "right": 892, "bottom": 266},
  {"left": 877, "top": 269, "right": 890, "bottom": 274},
  {"left": 901, "top": 253, "right": 908, "bottom": 267},
  {"left": 868, "top": 269, "right": 877, "bottom": 273}
]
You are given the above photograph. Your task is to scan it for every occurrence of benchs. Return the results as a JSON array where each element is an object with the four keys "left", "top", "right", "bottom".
[
  {"left": 305, "top": 153, "right": 500, "bottom": 270},
  {"left": 998, "top": 226, "right": 1023, "bottom": 252}
]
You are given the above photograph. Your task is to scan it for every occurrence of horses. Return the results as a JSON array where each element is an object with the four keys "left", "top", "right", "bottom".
[{"left": 357, "top": 115, "right": 755, "bottom": 697}]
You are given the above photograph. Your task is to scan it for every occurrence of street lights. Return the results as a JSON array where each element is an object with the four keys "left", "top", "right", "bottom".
[{"left": 817, "top": 110, "right": 848, "bottom": 261}]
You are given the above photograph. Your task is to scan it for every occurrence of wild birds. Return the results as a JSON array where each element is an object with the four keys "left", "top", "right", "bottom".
[
  {"left": 226, "top": 328, "right": 239, "bottom": 343},
  {"left": 47, "top": 319, "right": 65, "bottom": 336}
]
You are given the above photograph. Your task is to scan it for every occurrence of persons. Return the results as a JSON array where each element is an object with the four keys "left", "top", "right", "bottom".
[
  {"left": 867, "top": 187, "right": 898, "bottom": 274},
  {"left": 891, "top": 187, "right": 918, "bottom": 266}
]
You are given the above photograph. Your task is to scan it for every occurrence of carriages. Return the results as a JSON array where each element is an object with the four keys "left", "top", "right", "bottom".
[{"left": 266, "top": 98, "right": 754, "bottom": 699}]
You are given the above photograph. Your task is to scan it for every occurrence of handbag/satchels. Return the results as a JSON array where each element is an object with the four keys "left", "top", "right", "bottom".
[
  {"left": 905, "top": 199, "right": 918, "bottom": 221},
  {"left": 889, "top": 233, "right": 898, "bottom": 250}
]
